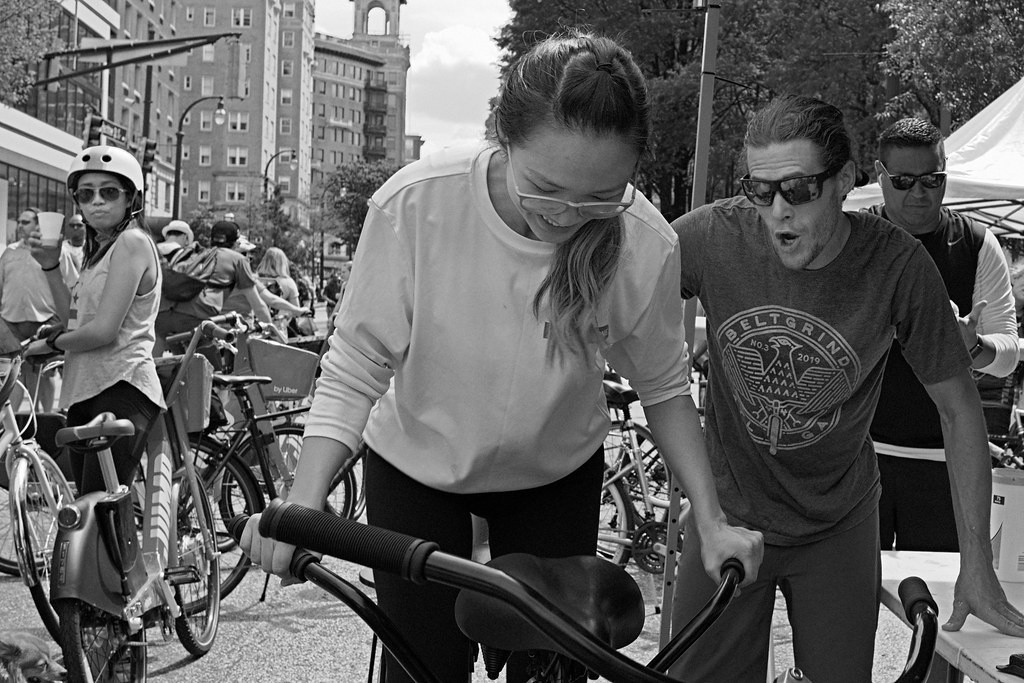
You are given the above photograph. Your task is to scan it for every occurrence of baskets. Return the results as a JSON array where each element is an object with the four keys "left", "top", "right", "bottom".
[
  {"left": 154, "top": 353, "right": 214, "bottom": 433},
  {"left": 247, "top": 337, "right": 320, "bottom": 401}
]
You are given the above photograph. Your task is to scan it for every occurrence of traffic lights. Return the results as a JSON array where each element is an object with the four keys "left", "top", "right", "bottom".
[
  {"left": 81, "top": 112, "right": 104, "bottom": 152},
  {"left": 137, "top": 137, "right": 157, "bottom": 191}
]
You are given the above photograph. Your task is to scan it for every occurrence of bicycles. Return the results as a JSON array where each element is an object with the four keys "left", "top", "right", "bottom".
[
  {"left": 289, "top": 543, "right": 744, "bottom": 683},
  {"left": 0, "top": 322, "right": 81, "bottom": 646},
  {"left": 49, "top": 319, "right": 235, "bottom": 683},
  {"left": 128, "top": 309, "right": 373, "bottom": 617},
  {"left": 988, "top": 409, "right": 1024, "bottom": 470},
  {"left": 258, "top": 495, "right": 938, "bottom": 683},
  {"left": 595, "top": 343, "right": 715, "bottom": 574}
]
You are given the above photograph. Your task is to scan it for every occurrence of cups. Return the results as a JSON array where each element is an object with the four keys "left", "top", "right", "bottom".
[{"left": 37, "top": 212, "right": 65, "bottom": 248}]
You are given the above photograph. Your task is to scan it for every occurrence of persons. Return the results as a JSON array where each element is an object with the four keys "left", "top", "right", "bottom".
[
  {"left": 321, "top": 260, "right": 353, "bottom": 320},
  {"left": 856, "top": 117, "right": 1019, "bottom": 554},
  {"left": 153, "top": 218, "right": 317, "bottom": 477},
  {"left": 22, "top": 146, "right": 168, "bottom": 496},
  {"left": 236, "top": 30, "right": 765, "bottom": 683},
  {"left": 671, "top": 95, "right": 1024, "bottom": 683},
  {"left": 0, "top": 208, "right": 88, "bottom": 484}
]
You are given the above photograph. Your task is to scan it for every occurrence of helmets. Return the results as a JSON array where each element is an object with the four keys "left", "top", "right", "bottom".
[{"left": 67, "top": 145, "right": 145, "bottom": 197}]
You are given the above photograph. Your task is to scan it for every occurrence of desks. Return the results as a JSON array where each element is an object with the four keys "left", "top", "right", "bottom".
[{"left": 880, "top": 550, "right": 1024, "bottom": 683}]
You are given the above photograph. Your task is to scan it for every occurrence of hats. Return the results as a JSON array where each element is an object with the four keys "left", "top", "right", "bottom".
[
  {"left": 210, "top": 221, "right": 240, "bottom": 247},
  {"left": 162, "top": 220, "right": 194, "bottom": 246},
  {"left": 233, "top": 236, "right": 257, "bottom": 252}
]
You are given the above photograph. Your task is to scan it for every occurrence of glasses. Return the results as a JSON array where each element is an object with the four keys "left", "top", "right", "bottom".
[
  {"left": 879, "top": 160, "right": 949, "bottom": 190},
  {"left": 18, "top": 220, "right": 31, "bottom": 226},
  {"left": 69, "top": 223, "right": 84, "bottom": 229},
  {"left": 740, "top": 162, "right": 844, "bottom": 207},
  {"left": 72, "top": 185, "right": 128, "bottom": 204},
  {"left": 505, "top": 135, "right": 641, "bottom": 220}
]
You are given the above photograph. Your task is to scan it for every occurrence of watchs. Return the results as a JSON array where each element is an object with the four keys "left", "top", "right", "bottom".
[
  {"left": 968, "top": 336, "right": 984, "bottom": 359},
  {"left": 46, "top": 330, "right": 64, "bottom": 351}
]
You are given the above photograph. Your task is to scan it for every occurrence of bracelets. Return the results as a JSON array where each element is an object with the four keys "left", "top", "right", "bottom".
[{"left": 42, "top": 262, "right": 60, "bottom": 272}]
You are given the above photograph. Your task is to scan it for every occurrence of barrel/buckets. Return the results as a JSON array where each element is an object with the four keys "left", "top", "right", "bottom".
[{"left": 990, "top": 468, "right": 1024, "bottom": 583}]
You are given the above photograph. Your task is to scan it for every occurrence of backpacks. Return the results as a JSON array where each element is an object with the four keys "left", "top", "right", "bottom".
[{"left": 160, "top": 241, "right": 236, "bottom": 303}]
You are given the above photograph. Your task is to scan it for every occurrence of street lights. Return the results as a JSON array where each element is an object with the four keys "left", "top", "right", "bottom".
[
  {"left": 171, "top": 95, "right": 226, "bottom": 221},
  {"left": 320, "top": 180, "right": 347, "bottom": 289},
  {"left": 263, "top": 150, "right": 298, "bottom": 234}
]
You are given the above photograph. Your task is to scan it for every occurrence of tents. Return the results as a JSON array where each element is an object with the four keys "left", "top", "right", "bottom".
[{"left": 841, "top": 73, "right": 1024, "bottom": 240}]
